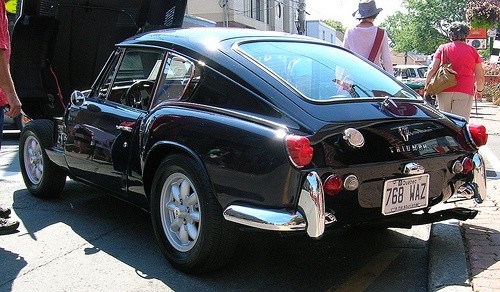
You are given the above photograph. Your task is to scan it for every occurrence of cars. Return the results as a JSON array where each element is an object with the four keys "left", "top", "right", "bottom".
[
  {"left": 3, "top": 108, "right": 62, "bottom": 137},
  {"left": 399, "top": 79, "right": 424, "bottom": 98}
]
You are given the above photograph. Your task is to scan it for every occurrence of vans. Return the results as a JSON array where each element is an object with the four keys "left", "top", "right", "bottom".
[
  {"left": 391, "top": 64, "right": 429, "bottom": 83},
  {"left": 12, "top": 0, "right": 490, "bottom": 274}
]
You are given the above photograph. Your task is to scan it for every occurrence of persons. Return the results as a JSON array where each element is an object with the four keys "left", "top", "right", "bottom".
[
  {"left": 0, "top": 0, "right": 22, "bottom": 237},
  {"left": 424, "top": 20, "right": 485, "bottom": 125},
  {"left": 342, "top": 0, "right": 395, "bottom": 79}
]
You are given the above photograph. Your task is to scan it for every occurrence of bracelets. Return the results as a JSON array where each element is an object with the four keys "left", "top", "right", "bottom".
[{"left": 476, "top": 89, "right": 484, "bottom": 93}]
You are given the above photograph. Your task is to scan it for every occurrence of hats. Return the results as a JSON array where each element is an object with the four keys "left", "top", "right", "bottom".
[{"left": 352, "top": 0, "right": 383, "bottom": 19}]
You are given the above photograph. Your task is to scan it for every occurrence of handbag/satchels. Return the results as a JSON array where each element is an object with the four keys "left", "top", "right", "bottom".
[{"left": 425, "top": 43, "right": 457, "bottom": 95}]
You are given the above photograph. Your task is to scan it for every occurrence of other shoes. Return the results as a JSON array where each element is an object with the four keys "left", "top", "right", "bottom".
[
  {"left": 0, "top": 217, "right": 19, "bottom": 235},
  {"left": 0, "top": 208, "right": 11, "bottom": 218}
]
